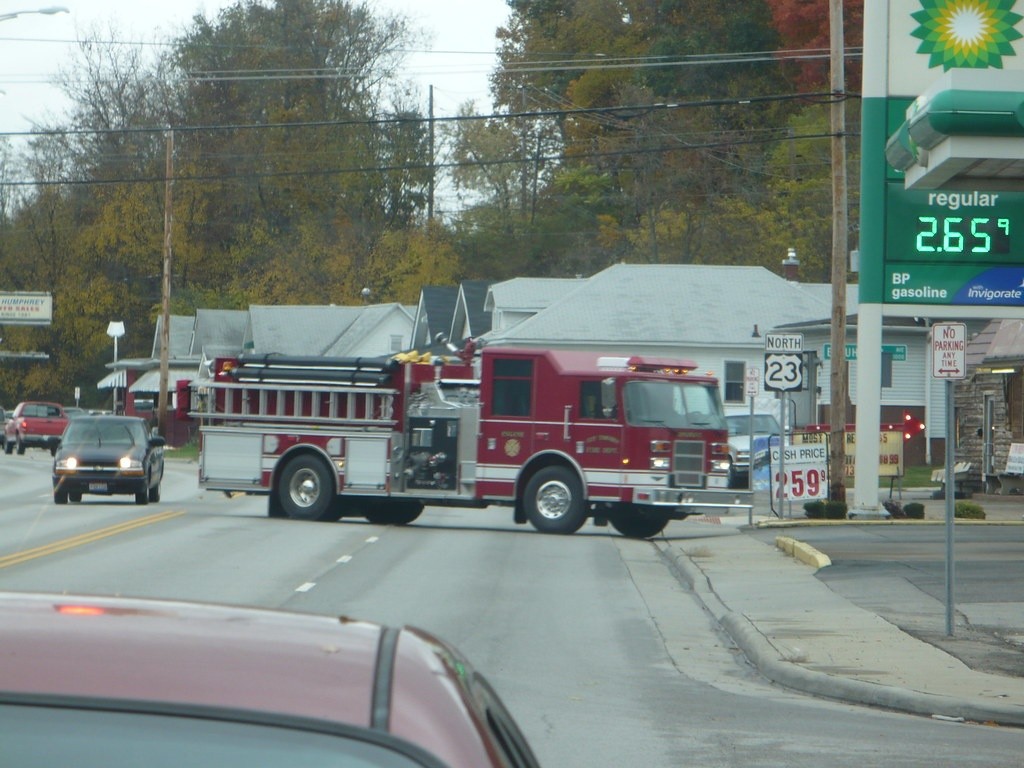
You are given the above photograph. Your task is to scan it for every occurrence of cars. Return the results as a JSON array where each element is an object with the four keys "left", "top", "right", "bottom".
[
  {"left": 713, "top": 411, "right": 787, "bottom": 475},
  {"left": 89, "top": 410, "right": 114, "bottom": 417},
  {"left": 61, "top": 407, "right": 84, "bottom": 420},
  {"left": 46, "top": 415, "right": 166, "bottom": 505},
  {"left": 1, "top": 594, "right": 542, "bottom": 768}
]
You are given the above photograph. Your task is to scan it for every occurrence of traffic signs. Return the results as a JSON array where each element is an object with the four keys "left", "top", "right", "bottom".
[{"left": 931, "top": 323, "right": 967, "bottom": 381}]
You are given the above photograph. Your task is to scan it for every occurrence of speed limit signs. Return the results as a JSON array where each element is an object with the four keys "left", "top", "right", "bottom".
[{"left": 764, "top": 352, "right": 804, "bottom": 394}]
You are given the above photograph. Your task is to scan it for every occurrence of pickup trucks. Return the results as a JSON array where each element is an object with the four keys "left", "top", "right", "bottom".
[{"left": 3, "top": 402, "right": 70, "bottom": 456}]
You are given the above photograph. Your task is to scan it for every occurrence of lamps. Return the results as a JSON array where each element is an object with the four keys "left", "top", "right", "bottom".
[{"left": 751, "top": 324, "right": 761, "bottom": 340}]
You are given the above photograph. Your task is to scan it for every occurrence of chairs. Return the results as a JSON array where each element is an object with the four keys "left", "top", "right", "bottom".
[
  {"left": 930, "top": 462, "right": 972, "bottom": 500},
  {"left": 80, "top": 430, "right": 100, "bottom": 440}
]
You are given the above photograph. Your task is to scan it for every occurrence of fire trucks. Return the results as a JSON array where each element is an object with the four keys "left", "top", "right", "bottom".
[{"left": 185, "top": 337, "right": 758, "bottom": 543}]
